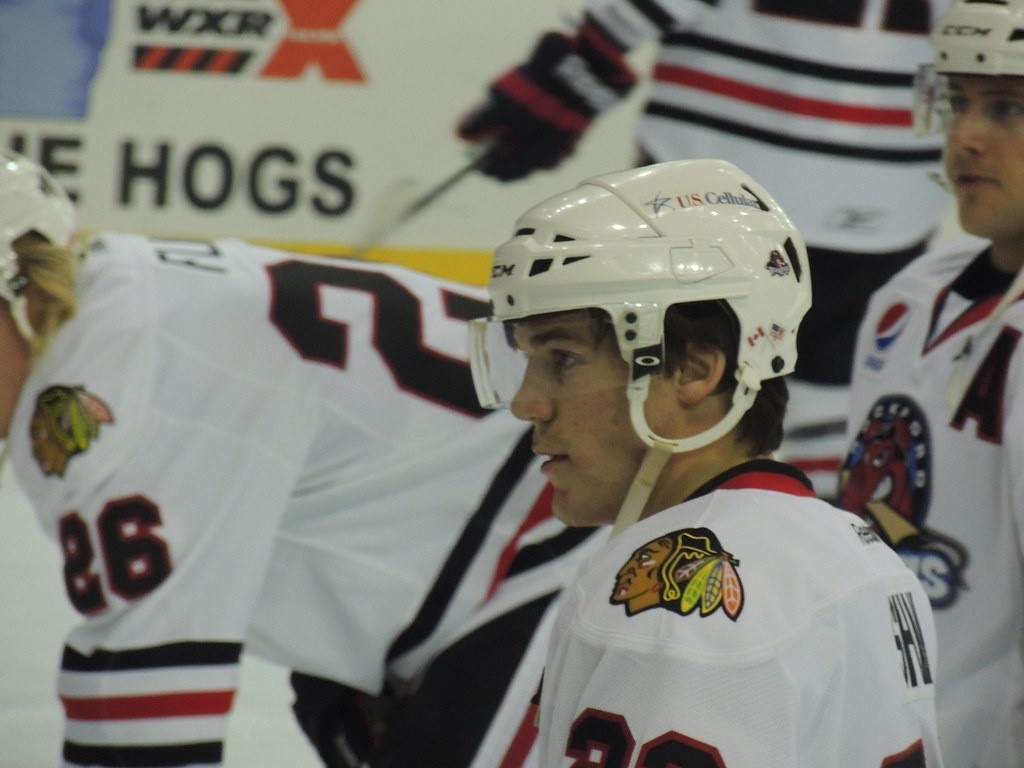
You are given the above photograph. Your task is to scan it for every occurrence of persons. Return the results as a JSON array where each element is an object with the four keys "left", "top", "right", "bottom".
[
  {"left": 840, "top": 0, "right": 1024, "bottom": 768},
  {"left": 468, "top": 159, "right": 944, "bottom": 768},
  {"left": 0, "top": 153, "right": 614, "bottom": 768},
  {"left": 454, "top": 1, "right": 953, "bottom": 386}
]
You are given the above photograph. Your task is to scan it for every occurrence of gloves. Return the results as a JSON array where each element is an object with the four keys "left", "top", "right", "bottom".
[{"left": 457, "top": 31, "right": 639, "bottom": 183}]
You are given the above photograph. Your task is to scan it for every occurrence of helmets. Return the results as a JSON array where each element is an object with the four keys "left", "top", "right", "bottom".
[
  {"left": 914, "top": 0, "right": 1024, "bottom": 138},
  {"left": 0, "top": 150, "right": 75, "bottom": 301},
  {"left": 468, "top": 156, "right": 813, "bottom": 411}
]
